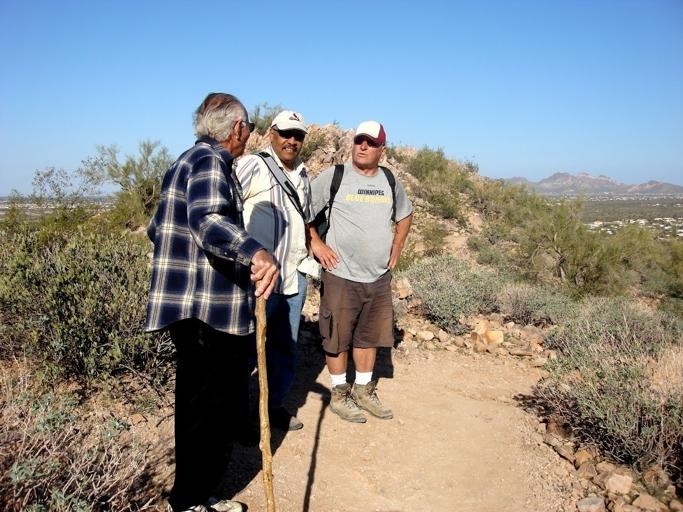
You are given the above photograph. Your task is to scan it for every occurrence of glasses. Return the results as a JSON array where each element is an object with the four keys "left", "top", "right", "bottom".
[
  {"left": 355, "top": 136, "right": 376, "bottom": 146},
  {"left": 242, "top": 121, "right": 254, "bottom": 132},
  {"left": 273, "top": 129, "right": 304, "bottom": 141}
]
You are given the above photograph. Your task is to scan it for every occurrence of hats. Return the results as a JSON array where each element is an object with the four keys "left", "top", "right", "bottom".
[
  {"left": 271, "top": 111, "right": 308, "bottom": 134},
  {"left": 356, "top": 120, "right": 385, "bottom": 143}
]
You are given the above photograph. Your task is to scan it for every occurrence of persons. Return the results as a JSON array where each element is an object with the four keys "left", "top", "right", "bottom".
[
  {"left": 233, "top": 108, "right": 316, "bottom": 448},
  {"left": 146, "top": 92, "right": 281, "bottom": 511},
  {"left": 301, "top": 121, "right": 414, "bottom": 423}
]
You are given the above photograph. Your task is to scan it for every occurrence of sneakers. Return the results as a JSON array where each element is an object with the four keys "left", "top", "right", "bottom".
[
  {"left": 330, "top": 383, "right": 367, "bottom": 422},
  {"left": 268, "top": 406, "right": 302, "bottom": 430},
  {"left": 167, "top": 498, "right": 243, "bottom": 512},
  {"left": 350, "top": 380, "right": 393, "bottom": 419}
]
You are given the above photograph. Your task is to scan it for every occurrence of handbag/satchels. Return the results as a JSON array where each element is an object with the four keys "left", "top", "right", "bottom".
[{"left": 301, "top": 275, "right": 321, "bottom": 322}]
